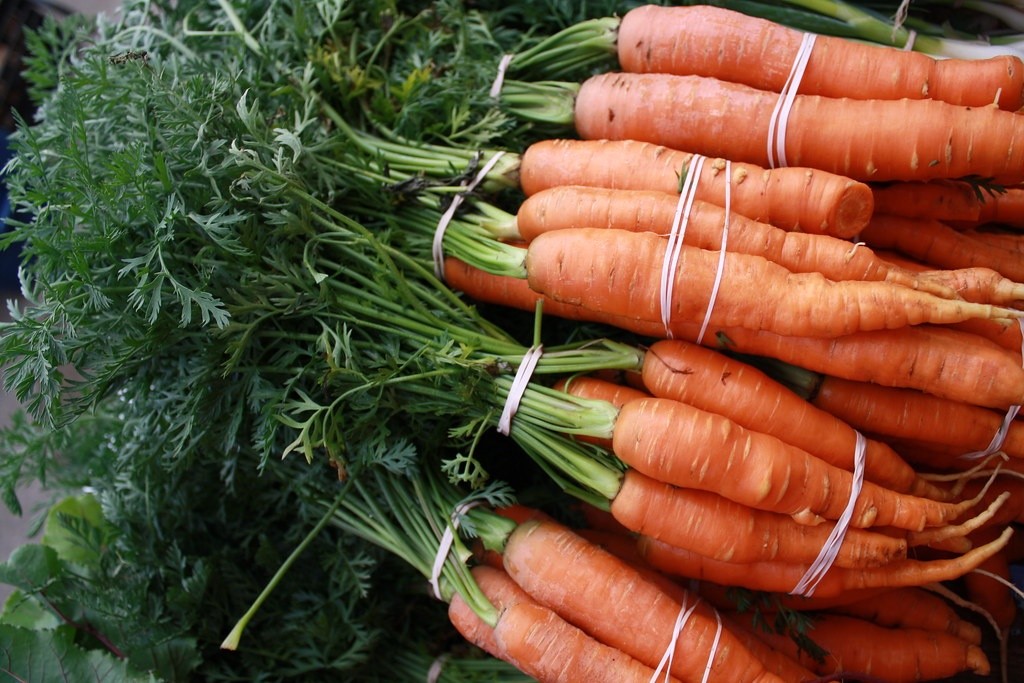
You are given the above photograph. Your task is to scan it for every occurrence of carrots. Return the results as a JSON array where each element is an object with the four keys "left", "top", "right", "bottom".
[{"left": 0, "top": 1, "right": 1024, "bottom": 682}]
